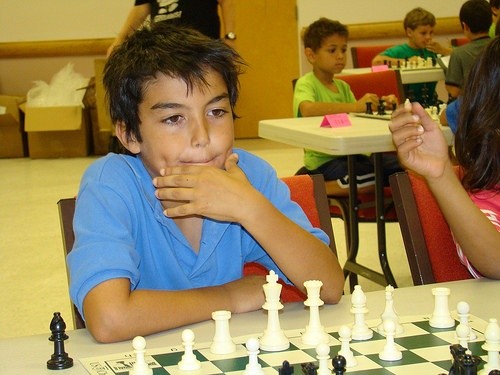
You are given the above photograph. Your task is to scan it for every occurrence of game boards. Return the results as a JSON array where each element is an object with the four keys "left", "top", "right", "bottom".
[
  {"left": 383, "top": 52, "right": 443, "bottom": 71},
  {"left": 78, "top": 269, "right": 500, "bottom": 375},
  {"left": 354, "top": 92, "right": 453, "bottom": 127}
]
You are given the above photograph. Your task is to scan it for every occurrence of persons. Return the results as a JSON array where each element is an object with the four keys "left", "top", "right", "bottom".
[
  {"left": 63, "top": 24, "right": 345, "bottom": 345},
  {"left": 444, "top": 0, "right": 493, "bottom": 97},
  {"left": 372, "top": 7, "right": 456, "bottom": 114},
  {"left": 294, "top": 18, "right": 405, "bottom": 187},
  {"left": 389, "top": 16, "right": 500, "bottom": 278},
  {"left": 489, "top": 0, "right": 500, "bottom": 38},
  {"left": 107, "top": 0, "right": 237, "bottom": 60}
]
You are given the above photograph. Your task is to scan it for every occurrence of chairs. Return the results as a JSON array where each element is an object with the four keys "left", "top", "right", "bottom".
[{"left": 57, "top": 36, "right": 475, "bottom": 330}]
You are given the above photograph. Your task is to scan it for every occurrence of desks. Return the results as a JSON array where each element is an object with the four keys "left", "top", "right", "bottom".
[
  {"left": 0, "top": 277, "right": 500, "bottom": 375},
  {"left": 260, "top": 107, "right": 455, "bottom": 290},
  {"left": 340, "top": 57, "right": 452, "bottom": 84}
]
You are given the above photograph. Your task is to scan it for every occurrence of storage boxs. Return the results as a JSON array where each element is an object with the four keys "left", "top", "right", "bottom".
[{"left": 0, "top": 58, "right": 112, "bottom": 159}]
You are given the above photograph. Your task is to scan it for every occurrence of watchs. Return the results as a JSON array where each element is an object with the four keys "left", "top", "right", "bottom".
[{"left": 225, "top": 32, "right": 236, "bottom": 40}]
